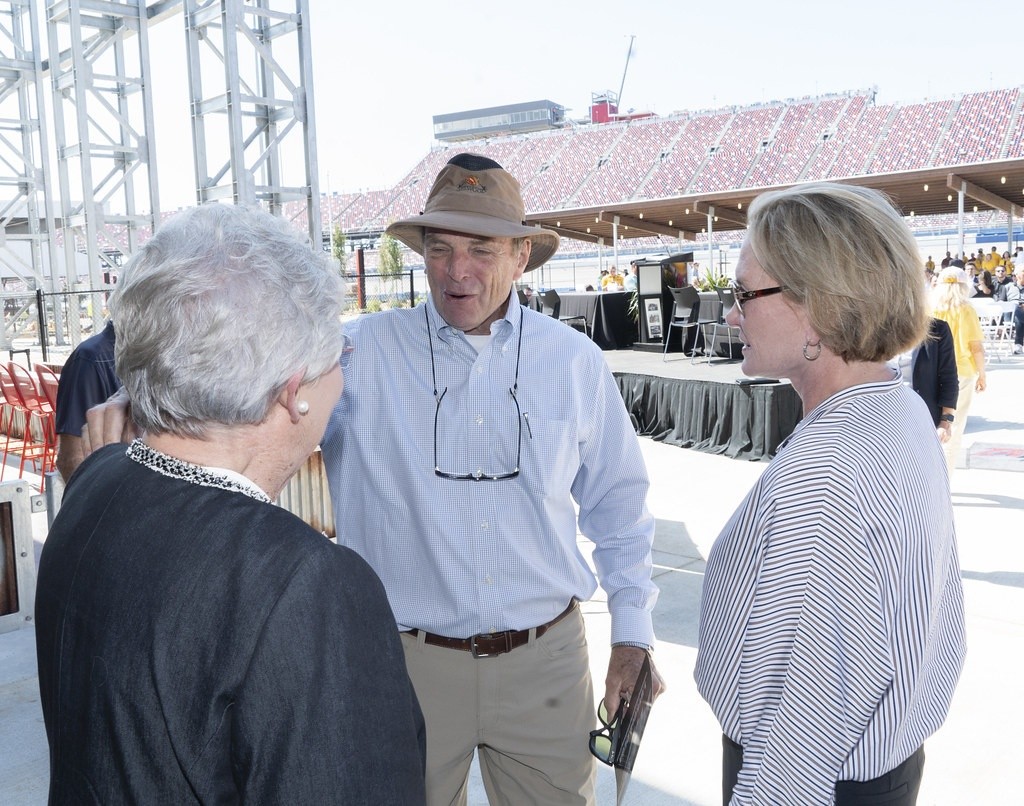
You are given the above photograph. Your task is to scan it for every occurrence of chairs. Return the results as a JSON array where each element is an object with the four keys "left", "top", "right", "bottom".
[
  {"left": 707, "top": 286, "right": 738, "bottom": 366},
  {"left": 8, "top": 362, "right": 56, "bottom": 478},
  {"left": 969, "top": 297, "right": 1018, "bottom": 364},
  {"left": 0, "top": 364, "right": 47, "bottom": 481},
  {"left": 663, "top": 286, "right": 717, "bottom": 364},
  {"left": 517, "top": 289, "right": 588, "bottom": 335},
  {"left": 34, "top": 362, "right": 59, "bottom": 494}
]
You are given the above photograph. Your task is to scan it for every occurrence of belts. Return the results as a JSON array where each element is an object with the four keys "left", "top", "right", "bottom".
[{"left": 405, "top": 597, "right": 575, "bottom": 659}]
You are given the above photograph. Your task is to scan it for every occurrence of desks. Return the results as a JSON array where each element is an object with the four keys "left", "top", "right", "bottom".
[
  {"left": 697, "top": 290, "right": 745, "bottom": 359},
  {"left": 0, "top": 371, "right": 60, "bottom": 449},
  {"left": 530, "top": 291, "right": 638, "bottom": 351}
]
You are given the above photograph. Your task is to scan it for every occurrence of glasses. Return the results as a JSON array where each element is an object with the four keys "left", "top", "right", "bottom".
[
  {"left": 434, "top": 387, "right": 521, "bottom": 482},
  {"left": 732, "top": 281, "right": 800, "bottom": 319},
  {"left": 589, "top": 697, "right": 626, "bottom": 766},
  {"left": 996, "top": 270, "right": 1005, "bottom": 272}
]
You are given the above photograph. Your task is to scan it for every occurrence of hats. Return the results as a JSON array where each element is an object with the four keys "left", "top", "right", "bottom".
[{"left": 385, "top": 152, "right": 559, "bottom": 274}]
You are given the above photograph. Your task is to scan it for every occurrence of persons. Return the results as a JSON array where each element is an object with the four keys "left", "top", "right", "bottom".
[
  {"left": 693, "top": 186, "right": 967, "bottom": 806},
  {"left": 888, "top": 266, "right": 986, "bottom": 442},
  {"left": 597, "top": 261, "right": 638, "bottom": 292},
  {"left": 33, "top": 203, "right": 426, "bottom": 806},
  {"left": 942, "top": 246, "right": 1024, "bottom": 355},
  {"left": 925, "top": 256, "right": 942, "bottom": 291},
  {"left": 81, "top": 153, "right": 665, "bottom": 806},
  {"left": 56, "top": 319, "right": 126, "bottom": 484},
  {"left": 687, "top": 262, "right": 701, "bottom": 291},
  {"left": 523, "top": 288, "right": 532, "bottom": 309}
]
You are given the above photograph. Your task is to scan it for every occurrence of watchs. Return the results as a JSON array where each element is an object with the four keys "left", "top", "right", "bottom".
[{"left": 941, "top": 414, "right": 954, "bottom": 422}]
